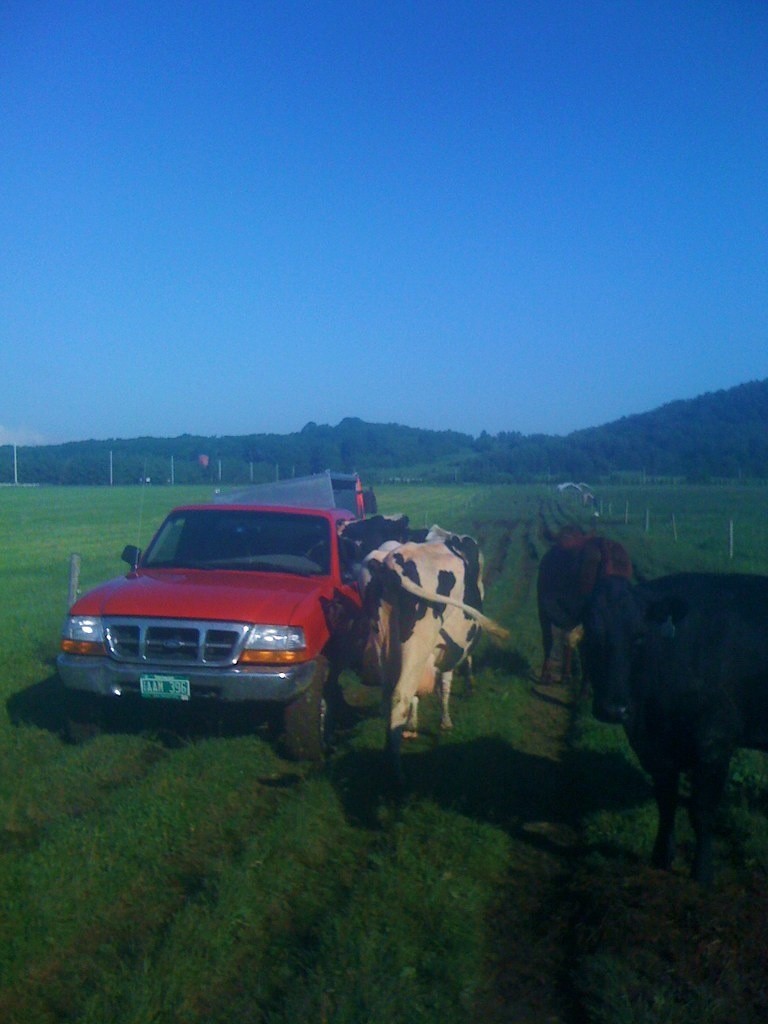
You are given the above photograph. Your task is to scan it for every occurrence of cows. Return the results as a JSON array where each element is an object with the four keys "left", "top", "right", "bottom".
[
  {"left": 582, "top": 571, "right": 768, "bottom": 885},
  {"left": 540, "top": 526, "right": 632, "bottom": 680},
  {"left": 337, "top": 515, "right": 510, "bottom": 782}
]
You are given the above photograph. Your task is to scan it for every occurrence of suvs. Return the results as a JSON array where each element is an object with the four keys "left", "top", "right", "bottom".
[{"left": 58, "top": 504, "right": 410, "bottom": 764}]
